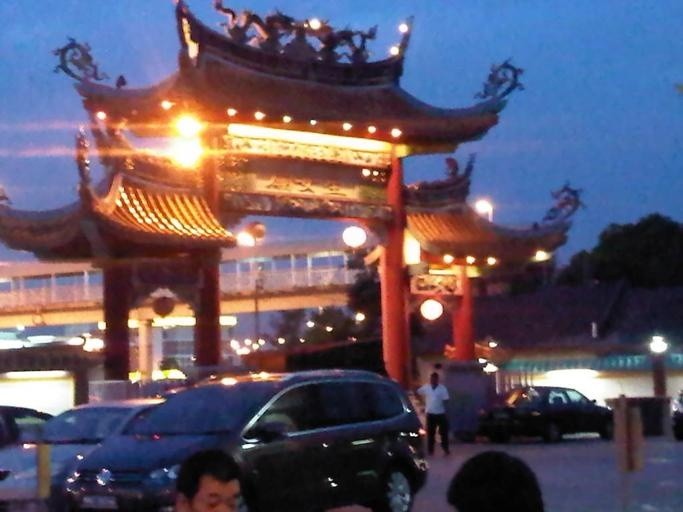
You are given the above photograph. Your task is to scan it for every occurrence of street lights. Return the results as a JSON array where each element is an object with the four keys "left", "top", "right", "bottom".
[
  {"left": 474, "top": 198, "right": 497, "bottom": 226},
  {"left": 237, "top": 219, "right": 267, "bottom": 344},
  {"left": 649, "top": 333, "right": 670, "bottom": 396}
]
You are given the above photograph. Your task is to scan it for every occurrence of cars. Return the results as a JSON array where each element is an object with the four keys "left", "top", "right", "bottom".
[
  {"left": 0, "top": 395, "right": 172, "bottom": 511},
  {"left": 472, "top": 383, "right": 616, "bottom": 445},
  {"left": 0, "top": 405, "right": 54, "bottom": 449}
]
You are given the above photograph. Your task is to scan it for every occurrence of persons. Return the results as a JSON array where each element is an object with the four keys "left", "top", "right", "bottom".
[
  {"left": 414, "top": 372, "right": 451, "bottom": 455},
  {"left": 175, "top": 448, "right": 239, "bottom": 511},
  {"left": 446, "top": 451, "right": 545, "bottom": 511}
]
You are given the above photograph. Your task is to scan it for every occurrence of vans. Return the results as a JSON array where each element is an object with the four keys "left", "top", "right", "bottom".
[{"left": 65, "top": 367, "right": 435, "bottom": 511}]
